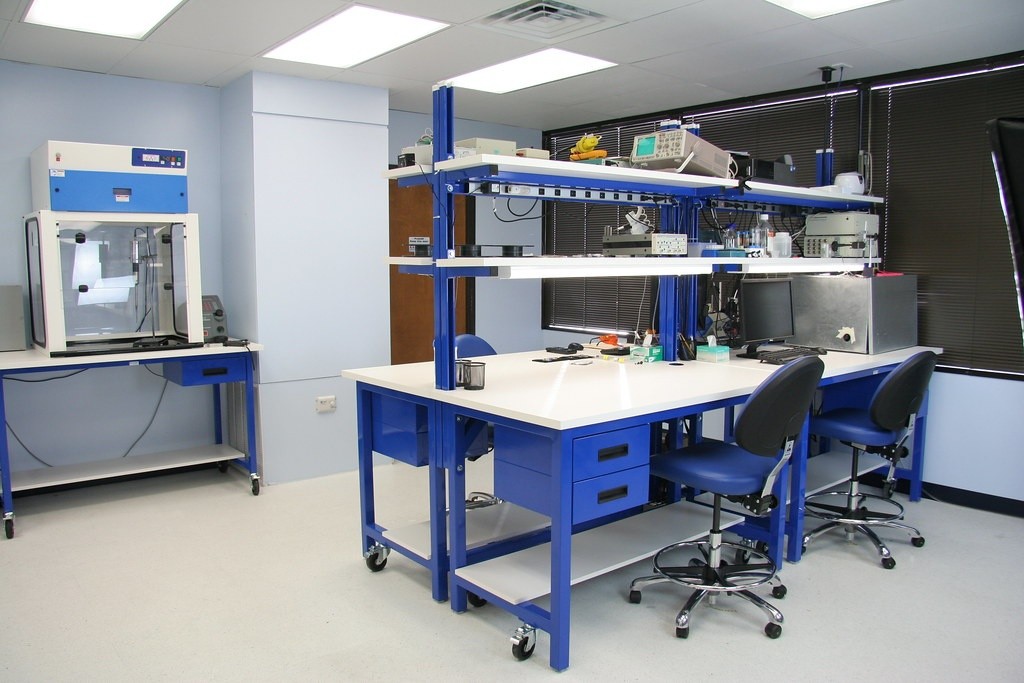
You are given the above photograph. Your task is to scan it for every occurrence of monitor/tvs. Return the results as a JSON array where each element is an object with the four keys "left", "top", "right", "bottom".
[
  {"left": 649, "top": 274, "right": 708, "bottom": 334},
  {"left": 736, "top": 278, "right": 795, "bottom": 360}
]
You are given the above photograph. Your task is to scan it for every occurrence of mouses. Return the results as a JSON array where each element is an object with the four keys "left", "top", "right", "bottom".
[
  {"left": 811, "top": 347, "right": 827, "bottom": 355},
  {"left": 568, "top": 343, "right": 583, "bottom": 350}
]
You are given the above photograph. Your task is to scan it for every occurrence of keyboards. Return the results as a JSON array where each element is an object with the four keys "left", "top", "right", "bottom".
[
  {"left": 757, "top": 347, "right": 819, "bottom": 365},
  {"left": 600, "top": 347, "right": 630, "bottom": 355}
]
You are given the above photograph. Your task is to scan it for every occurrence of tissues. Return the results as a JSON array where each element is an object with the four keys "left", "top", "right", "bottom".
[
  {"left": 629, "top": 333, "right": 663, "bottom": 363},
  {"left": 696, "top": 334, "right": 731, "bottom": 364}
]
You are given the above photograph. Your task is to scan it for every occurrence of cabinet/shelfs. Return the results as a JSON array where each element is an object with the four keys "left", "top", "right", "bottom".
[
  {"left": 439, "top": 87, "right": 885, "bottom": 389},
  {"left": 387, "top": 87, "right": 823, "bottom": 389}
]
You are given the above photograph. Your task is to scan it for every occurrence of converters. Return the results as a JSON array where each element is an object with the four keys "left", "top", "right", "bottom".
[{"left": 398, "top": 153, "right": 415, "bottom": 167}]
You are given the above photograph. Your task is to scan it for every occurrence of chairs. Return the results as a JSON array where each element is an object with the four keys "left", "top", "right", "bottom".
[
  {"left": 445, "top": 334, "right": 504, "bottom": 516},
  {"left": 628, "top": 356, "right": 825, "bottom": 638},
  {"left": 802, "top": 350, "right": 936, "bottom": 570}
]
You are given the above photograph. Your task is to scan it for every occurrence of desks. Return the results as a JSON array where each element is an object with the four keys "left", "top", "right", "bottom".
[
  {"left": 342, "top": 344, "right": 945, "bottom": 671},
  {"left": 0, "top": 342, "right": 264, "bottom": 539}
]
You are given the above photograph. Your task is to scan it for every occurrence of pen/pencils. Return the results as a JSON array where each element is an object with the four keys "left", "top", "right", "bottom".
[{"left": 678, "top": 332, "right": 696, "bottom": 360}]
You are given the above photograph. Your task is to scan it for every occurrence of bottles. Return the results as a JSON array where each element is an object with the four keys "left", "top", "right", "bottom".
[{"left": 723, "top": 214, "right": 775, "bottom": 249}]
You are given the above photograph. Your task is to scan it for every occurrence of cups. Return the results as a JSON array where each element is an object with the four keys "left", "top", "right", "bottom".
[{"left": 455, "top": 360, "right": 486, "bottom": 389}]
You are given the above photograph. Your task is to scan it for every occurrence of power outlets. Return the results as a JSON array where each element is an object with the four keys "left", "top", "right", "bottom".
[{"left": 316, "top": 396, "right": 336, "bottom": 414}]
